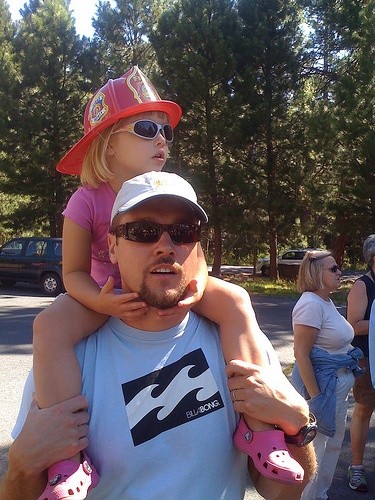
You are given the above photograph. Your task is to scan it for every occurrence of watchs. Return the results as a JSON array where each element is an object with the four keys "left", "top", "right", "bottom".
[{"left": 277, "top": 410, "right": 318, "bottom": 446}]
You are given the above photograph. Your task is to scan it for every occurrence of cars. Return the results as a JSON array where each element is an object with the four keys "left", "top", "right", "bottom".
[{"left": 257, "top": 249, "right": 308, "bottom": 276}]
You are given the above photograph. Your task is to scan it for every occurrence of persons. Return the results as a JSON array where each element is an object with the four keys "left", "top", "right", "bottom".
[
  {"left": 346, "top": 234, "right": 375, "bottom": 491},
  {"left": 33, "top": 65, "right": 304, "bottom": 500},
  {"left": 0, "top": 170, "right": 318, "bottom": 500},
  {"left": 291, "top": 250, "right": 366, "bottom": 500}
]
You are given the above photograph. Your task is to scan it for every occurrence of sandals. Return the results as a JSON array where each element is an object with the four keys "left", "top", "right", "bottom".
[
  {"left": 37, "top": 450, "right": 100, "bottom": 500},
  {"left": 233, "top": 416, "right": 304, "bottom": 486}
]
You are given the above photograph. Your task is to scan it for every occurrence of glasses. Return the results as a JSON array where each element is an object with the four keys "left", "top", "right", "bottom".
[
  {"left": 110, "top": 119, "right": 174, "bottom": 142},
  {"left": 108, "top": 219, "right": 202, "bottom": 244},
  {"left": 328, "top": 265, "right": 339, "bottom": 274}
]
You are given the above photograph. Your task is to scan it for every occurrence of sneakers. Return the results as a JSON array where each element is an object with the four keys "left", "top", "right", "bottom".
[{"left": 347, "top": 464, "right": 368, "bottom": 491}]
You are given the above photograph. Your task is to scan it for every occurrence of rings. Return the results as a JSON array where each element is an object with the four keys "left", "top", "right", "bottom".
[{"left": 233, "top": 390, "right": 237, "bottom": 400}]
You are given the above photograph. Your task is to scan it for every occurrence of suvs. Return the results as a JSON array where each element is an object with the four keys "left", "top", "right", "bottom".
[{"left": 0, "top": 236, "right": 66, "bottom": 297}]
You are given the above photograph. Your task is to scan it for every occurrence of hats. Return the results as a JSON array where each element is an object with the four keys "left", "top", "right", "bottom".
[
  {"left": 110, "top": 171, "right": 209, "bottom": 226},
  {"left": 56, "top": 65, "right": 182, "bottom": 175}
]
